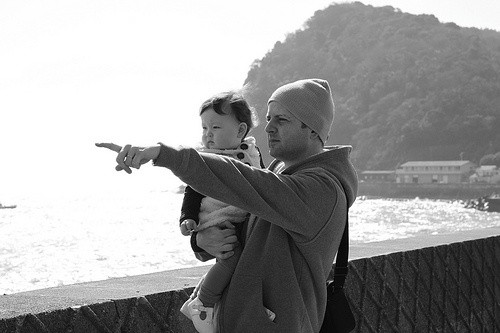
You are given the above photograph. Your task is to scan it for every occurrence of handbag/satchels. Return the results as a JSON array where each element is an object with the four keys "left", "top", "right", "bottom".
[{"left": 319, "top": 279, "right": 356, "bottom": 333}]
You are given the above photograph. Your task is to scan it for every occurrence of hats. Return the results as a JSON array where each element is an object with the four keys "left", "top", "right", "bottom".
[{"left": 267, "top": 78, "right": 335, "bottom": 145}]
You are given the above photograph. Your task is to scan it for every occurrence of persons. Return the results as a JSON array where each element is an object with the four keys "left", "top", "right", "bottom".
[{"left": 94, "top": 78, "right": 359, "bottom": 333}]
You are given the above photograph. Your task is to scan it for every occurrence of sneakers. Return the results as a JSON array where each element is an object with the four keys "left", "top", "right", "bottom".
[{"left": 180, "top": 297, "right": 224, "bottom": 333}]
